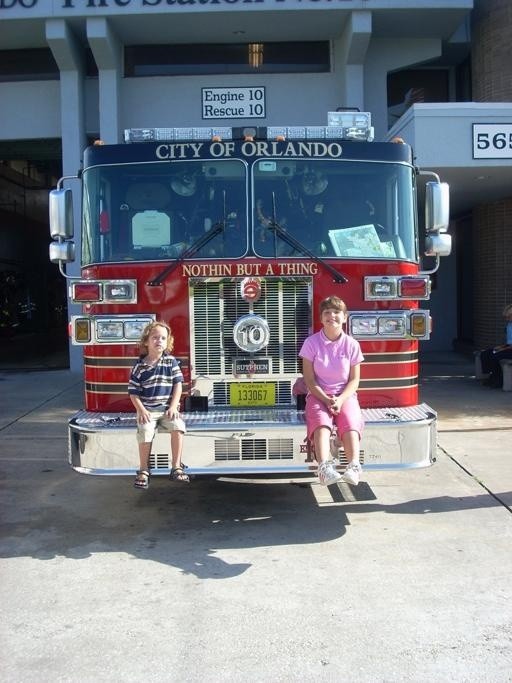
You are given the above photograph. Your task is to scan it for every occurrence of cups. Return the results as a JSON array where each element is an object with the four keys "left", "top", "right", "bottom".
[{"left": 166, "top": 241, "right": 187, "bottom": 258}]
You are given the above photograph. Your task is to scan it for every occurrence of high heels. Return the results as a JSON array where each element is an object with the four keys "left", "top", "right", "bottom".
[{"left": 482, "top": 372, "right": 503, "bottom": 388}]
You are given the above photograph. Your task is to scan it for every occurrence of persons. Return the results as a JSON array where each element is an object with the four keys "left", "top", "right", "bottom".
[
  {"left": 298, "top": 294, "right": 365, "bottom": 487},
  {"left": 127, "top": 321, "right": 191, "bottom": 490},
  {"left": 480, "top": 304, "right": 512, "bottom": 390}
]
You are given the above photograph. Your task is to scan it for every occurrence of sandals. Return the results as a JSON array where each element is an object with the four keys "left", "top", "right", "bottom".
[
  {"left": 133, "top": 469, "right": 151, "bottom": 489},
  {"left": 168, "top": 467, "right": 190, "bottom": 484}
]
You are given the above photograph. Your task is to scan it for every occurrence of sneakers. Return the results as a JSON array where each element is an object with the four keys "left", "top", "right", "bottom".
[
  {"left": 342, "top": 460, "right": 362, "bottom": 485},
  {"left": 317, "top": 460, "right": 341, "bottom": 485}
]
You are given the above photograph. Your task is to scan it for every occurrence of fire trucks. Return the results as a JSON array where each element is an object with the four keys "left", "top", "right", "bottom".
[{"left": 44, "top": 105, "right": 454, "bottom": 487}]
